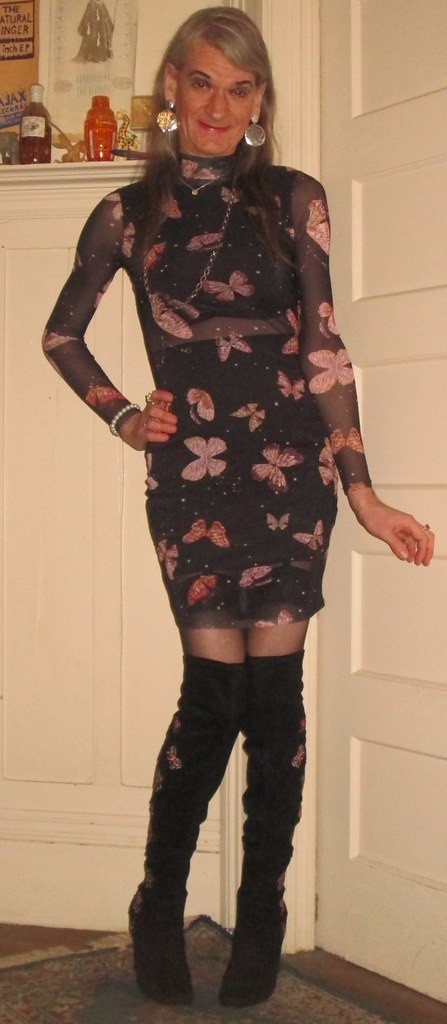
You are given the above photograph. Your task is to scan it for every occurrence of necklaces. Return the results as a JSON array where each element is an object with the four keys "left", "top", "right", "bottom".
[
  {"left": 143, "top": 188, "right": 236, "bottom": 311},
  {"left": 179, "top": 171, "right": 225, "bottom": 195}
]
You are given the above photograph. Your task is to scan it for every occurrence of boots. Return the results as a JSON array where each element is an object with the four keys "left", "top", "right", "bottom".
[
  {"left": 131, "top": 654, "right": 250, "bottom": 1004},
  {"left": 220, "top": 649, "right": 307, "bottom": 1008}
]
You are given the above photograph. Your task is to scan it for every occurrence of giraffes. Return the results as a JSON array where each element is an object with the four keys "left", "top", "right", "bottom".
[{"left": 114, "top": 109, "right": 140, "bottom": 150}]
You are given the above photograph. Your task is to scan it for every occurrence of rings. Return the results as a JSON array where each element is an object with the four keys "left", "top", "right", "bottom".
[
  {"left": 422, "top": 524, "right": 431, "bottom": 531},
  {"left": 145, "top": 392, "right": 154, "bottom": 405},
  {"left": 143, "top": 420, "right": 149, "bottom": 431}
]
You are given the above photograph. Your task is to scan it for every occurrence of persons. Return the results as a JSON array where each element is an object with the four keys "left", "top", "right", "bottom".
[{"left": 41, "top": 6, "right": 435, "bottom": 1009}]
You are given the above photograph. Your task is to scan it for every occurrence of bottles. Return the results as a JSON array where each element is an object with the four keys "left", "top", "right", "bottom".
[
  {"left": 84, "top": 95, "right": 118, "bottom": 162},
  {"left": 19, "top": 83, "right": 52, "bottom": 165}
]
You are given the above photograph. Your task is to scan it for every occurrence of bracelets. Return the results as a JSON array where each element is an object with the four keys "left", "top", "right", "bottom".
[{"left": 109, "top": 404, "right": 140, "bottom": 437}]
